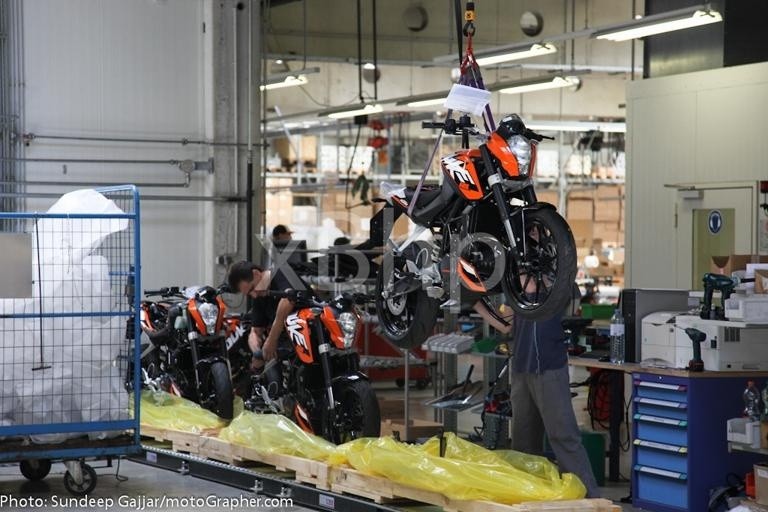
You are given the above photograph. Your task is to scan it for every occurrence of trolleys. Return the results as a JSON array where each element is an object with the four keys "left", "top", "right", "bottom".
[
  {"left": 266, "top": 241, "right": 437, "bottom": 391},
  {"left": 0, "top": 183, "right": 148, "bottom": 497}
]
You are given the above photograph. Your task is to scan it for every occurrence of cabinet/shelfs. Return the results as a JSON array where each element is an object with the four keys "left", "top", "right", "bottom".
[{"left": 726, "top": 441, "right": 768, "bottom": 512}]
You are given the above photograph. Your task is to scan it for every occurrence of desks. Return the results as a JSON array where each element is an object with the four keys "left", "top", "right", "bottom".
[{"left": 496, "top": 350, "right": 767, "bottom": 511}]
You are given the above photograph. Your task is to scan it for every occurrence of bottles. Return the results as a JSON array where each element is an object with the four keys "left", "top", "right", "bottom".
[
  {"left": 610, "top": 310, "right": 626, "bottom": 365},
  {"left": 743, "top": 380, "right": 761, "bottom": 417},
  {"left": 761, "top": 385, "right": 768, "bottom": 415}
]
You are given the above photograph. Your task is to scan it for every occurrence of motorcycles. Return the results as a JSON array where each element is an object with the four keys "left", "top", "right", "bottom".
[
  {"left": 243, "top": 287, "right": 385, "bottom": 444},
  {"left": 119, "top": 284, "right": 243, "bottom": 422},
  {"left": 222, "top": 308, "right": 253, "bottom": 401},
  {"left": 368, "top": 110, "right": 576, "bottom": 349}
]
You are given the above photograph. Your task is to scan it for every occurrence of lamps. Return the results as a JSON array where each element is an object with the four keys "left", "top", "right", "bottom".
[{"left": 257, "top": 1, "right": 727, "bottom": 132}]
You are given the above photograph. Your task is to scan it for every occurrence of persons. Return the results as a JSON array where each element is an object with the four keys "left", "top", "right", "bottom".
[
  {"left": 228, "top": 261, "right": 323, "bottom": 369},
  {"left": 273, "top": 225, "right": 292, "bottom": 240},
  {"left": 334, "top": 238, "right": 350, "bottom": 246},
  {"left": 473, "top": 227, "right": 602, "bottom": 498}
]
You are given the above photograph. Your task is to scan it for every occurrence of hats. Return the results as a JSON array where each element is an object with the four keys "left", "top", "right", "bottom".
[{"left": 272, "top": 225, "right": 294, "bottom": 235}]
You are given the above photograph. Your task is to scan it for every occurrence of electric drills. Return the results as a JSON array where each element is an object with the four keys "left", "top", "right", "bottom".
[{"left": 673, "top": 325, "right": 706, "bottom": 371}]
